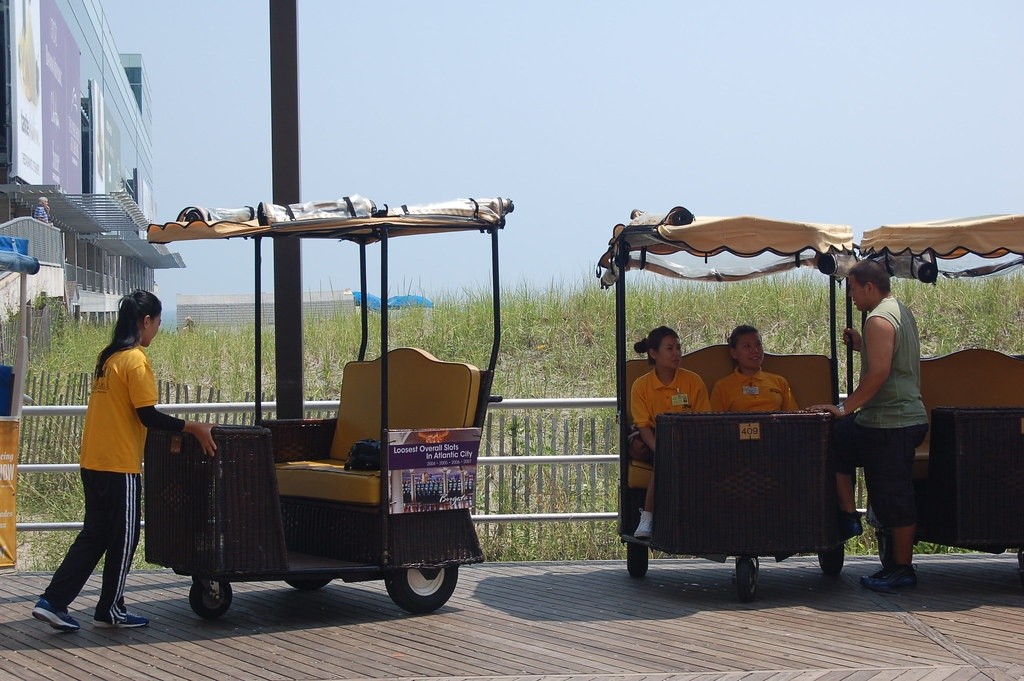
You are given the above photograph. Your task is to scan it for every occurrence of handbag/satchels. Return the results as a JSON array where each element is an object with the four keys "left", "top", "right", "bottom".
[
  {"left": 344, "top": 436, "right": 381, "bottom": 472},
  {"left": 626, "top": 423, "right": 655, "bottom": 464}
]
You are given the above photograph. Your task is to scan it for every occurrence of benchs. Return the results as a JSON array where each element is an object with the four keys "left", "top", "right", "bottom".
[
  {"left": 914, "top": 349, "right": 1024, "bottom": 482},
  {"left": 274, "top": 347, "right": 481, "bottom": 506},
  {"left": 625, "top": 345, "right": 833, "bottom": 487}
]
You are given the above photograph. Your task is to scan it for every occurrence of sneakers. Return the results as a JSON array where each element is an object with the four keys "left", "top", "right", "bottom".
[
  {"left": 860, "top": 558, "right": 919, "bottom": 593},
  {"left": 92, "top": 610, "right": 149, "bottom": 629},
  {"left": 833, "top": 510, "right": 864, "bottom": 542},
  {"left": 634, "top": 507, "right": 654, "bottom": 538},
  {"left": 32, "top": 596, "right": 80, "bottom": 631}
]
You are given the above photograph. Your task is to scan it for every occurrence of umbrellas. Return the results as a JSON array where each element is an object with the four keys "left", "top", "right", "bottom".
[
  {"left": 388, "top": 295, "right": 432, "bottom": 309},
  {"left": 352, "top": 292, "right": 383, "bottom": 312}
]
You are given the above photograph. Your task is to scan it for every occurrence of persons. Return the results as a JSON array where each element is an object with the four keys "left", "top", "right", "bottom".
[
  {"left": 805, "top": 260, "right": 928, "bottom": 591},
  {"left": 32, "top": 289, "right": 221, "bottom": 630},
  {"left": 626, "top": 326, "right": 710, "bottom": 538},
  {"left": 710, "top": 324, "right": 800, "bottom": 412},
  {"left": 34, "top": 197, "right": 53, "bottom": 227},
  {"left": 183, "top": 316, "right": 194, "bottom": 330}
]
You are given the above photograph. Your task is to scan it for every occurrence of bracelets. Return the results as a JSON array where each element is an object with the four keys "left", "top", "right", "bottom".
[{"left": 837, "top": 402, "right": 845, "bottom": 416}]
